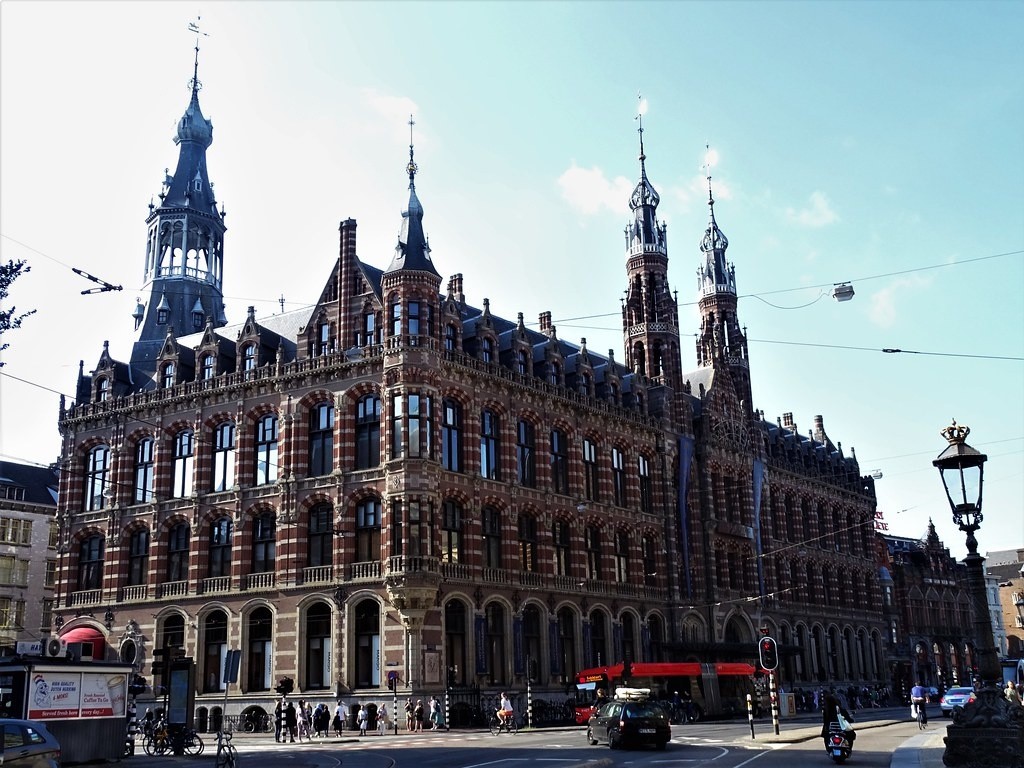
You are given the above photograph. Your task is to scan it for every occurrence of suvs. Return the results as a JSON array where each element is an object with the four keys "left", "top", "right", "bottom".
[{"left": 586, "top": 686, "right": 672, "bottom": 751}]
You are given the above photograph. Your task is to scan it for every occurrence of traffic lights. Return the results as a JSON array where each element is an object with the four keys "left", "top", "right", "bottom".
[{"left": 759, "top": 637, "right": 780, "bottom": 671}]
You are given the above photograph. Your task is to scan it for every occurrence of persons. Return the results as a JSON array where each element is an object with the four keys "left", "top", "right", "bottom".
[
  {"left": 773, "top": 681, "right": 893, "bottom": 714},
  {"left": 911, "top": 681, "right": 930, "bottom": 725},
  {"left": 428, "top": 695, "right": 439, "bottom": 730},
  {"left": 139, "top": 708, "right": 154, "bottom": 739},
  {"left": 272, "top": 698, "right": 346, "bottom": 743},
  {"left": 414, "top": 700, "right": 424, "bottom": 732},
  {"left": 1004, "top": 680, "right": 1022, "bottom": 707},
  {"left": 434, "top": 699, "right": 446, "bottom": 729},
  {"left": 821, "top": 696, "right": 856, "bottom": 754},
  {"left": 404, "top": 698, "right": 414, "bottom": 731},
  {"left": 589, "top": 688, "right": 695, "bottom": 725},
  {"left": 836, "top": 699, "right": 856, "bottom": 754},
  {"left": 497, "top": 692, "right": 514, "bottom": 725},
  {"left": 356, "top": 704, "right": 368, "bottom": 736},
  {"left": 377, "top": 703, "right": 387, "bottom": 735}
]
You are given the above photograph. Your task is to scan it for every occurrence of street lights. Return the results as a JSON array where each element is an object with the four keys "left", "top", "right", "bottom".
[{"left": 930, "top": 415, "right": 1024, "bottom": 768}]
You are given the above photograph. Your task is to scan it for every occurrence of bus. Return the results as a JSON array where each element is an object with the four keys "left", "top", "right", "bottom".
[{"left": 565, "top": 661, "right": 778, "bottom": 725}]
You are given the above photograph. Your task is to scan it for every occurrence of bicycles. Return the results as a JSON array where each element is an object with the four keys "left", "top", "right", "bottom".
[
  {"left": 213, "top": 727, "right": 239, "bottom": 768},
  {"left": 133, "top": 713, "right": 204, "bottom": 757},
  {"left": 243, "top": 714, "right": 276, "bottom": 734},
  {"left": 489, "top": 706, "right": 519, "bottom": 736},
  {"left": 911, "top": 702, "right": 927, "bottom": 730}
]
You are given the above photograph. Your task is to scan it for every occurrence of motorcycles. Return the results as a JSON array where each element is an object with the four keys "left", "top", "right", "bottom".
[{"left": 826, "top": 709, "right": 857, "bottom": 763}]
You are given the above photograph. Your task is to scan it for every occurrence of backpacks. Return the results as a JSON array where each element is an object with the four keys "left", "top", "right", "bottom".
[{"left": 433, "top": 701, "right": 440, "bottom": 712}]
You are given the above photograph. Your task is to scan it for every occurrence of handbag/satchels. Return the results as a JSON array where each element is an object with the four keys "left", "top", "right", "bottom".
[
  {"left": 911, "top": 702, "right": 919, "bottom": 719},
  {"left": 836, "top": 705, "right": 853, "bottom": 731},
  {"left": 434, "top": 712, "right": 444, "bottom": 724}
]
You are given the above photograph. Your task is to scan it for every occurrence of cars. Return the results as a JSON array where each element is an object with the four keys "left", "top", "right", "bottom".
[
  {"left": 0, "top": 718, "right": 62, "bottom": 768},
  {"left": 940, "top": 687, "right": 975, "bottom": 718},
  {"left": 924, "top": 687, "right": 941, "bottom": 702}
]
